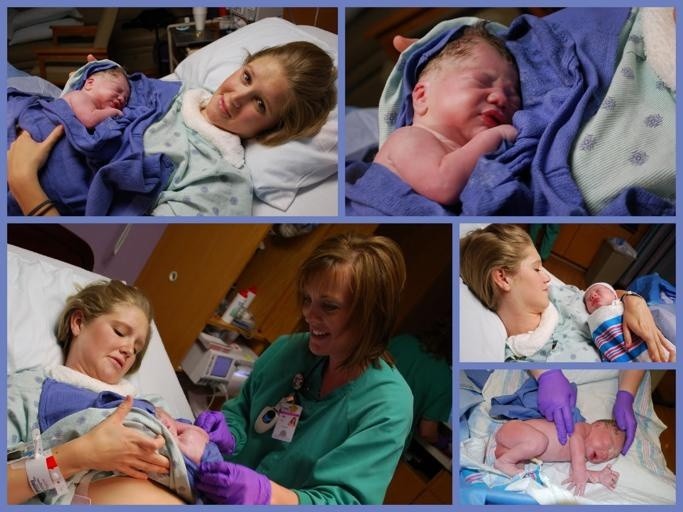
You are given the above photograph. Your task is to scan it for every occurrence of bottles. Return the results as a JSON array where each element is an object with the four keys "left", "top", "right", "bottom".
[{"left": 221, "top": 283, "right": 258, "bottom": 324}]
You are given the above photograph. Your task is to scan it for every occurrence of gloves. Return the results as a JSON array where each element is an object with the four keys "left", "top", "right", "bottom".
[
  {"left": 613, "top": 389, "right": 637, "bottom": 456},
  {"left": 194, "top": 408, "right": 237, "bottom": 456},
  {"left": 198, "top": 460, "right": 273, "bottom": 506},
  {"left": 534, "top": 369, "right": 577, "bottom": 445}
]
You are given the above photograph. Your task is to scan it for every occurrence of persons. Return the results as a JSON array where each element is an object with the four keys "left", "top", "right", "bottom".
[
  {"left": 492, "top": 417, "right": 626, "bottom": 497},
  {"left": 7, "top": 278, "right": 204, "bottom": 504},
  {"left": 8, "top": 41, "right": 336, "bottom": 216},
  {"left": 457, "top": 223, "right": 676, "bottom": 365},
  {"left": 391, "top": 34, "right": 419, "bottom": 54},
  {"left": 154, "top": 406, "right": 209, "bottom": 465},
  {"left": 195, "top": 237, "right": 413, "bottom": 505},
  {"left": 386, "top": 311, "right": 452, "bottom": 442},
  {"left": 525, "top": 369, "right": 651, "bottom": 456},
  {"left": 61, "top": 66, "right": 131, "bottom": 129},
  {"left": 582, "top": 279, "right": 654, "bottom": 362},
  {"left": 372, "top": 27, "right": 521, "bottom": 209}
]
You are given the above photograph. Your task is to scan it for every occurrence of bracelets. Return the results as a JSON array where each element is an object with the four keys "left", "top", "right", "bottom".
[
  {"left": 27, "top": 197, "right": 49, "bottom": 216},
  {"left": 36, "top": 202, "right": 53, "bottom": 216},
  {"left": 619, "top": 290, "right": 643, "bottom": 303}
]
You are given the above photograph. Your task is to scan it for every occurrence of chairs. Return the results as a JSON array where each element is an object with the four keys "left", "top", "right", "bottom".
[{"left": 33, "top": 24, "right": 108, "bottom": 89}]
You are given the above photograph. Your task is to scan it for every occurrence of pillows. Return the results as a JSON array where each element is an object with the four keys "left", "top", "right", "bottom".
[{"left": 178, "top": 16, "right": 341, "bottom": 207}]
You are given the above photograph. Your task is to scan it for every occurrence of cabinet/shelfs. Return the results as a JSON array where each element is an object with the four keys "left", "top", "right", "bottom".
[
  {"left": 132, "top": 223, "right": 378, "bottom": 376},
  {"left": 552, "top": 222, "right": 644, "bottom": 271}
]
